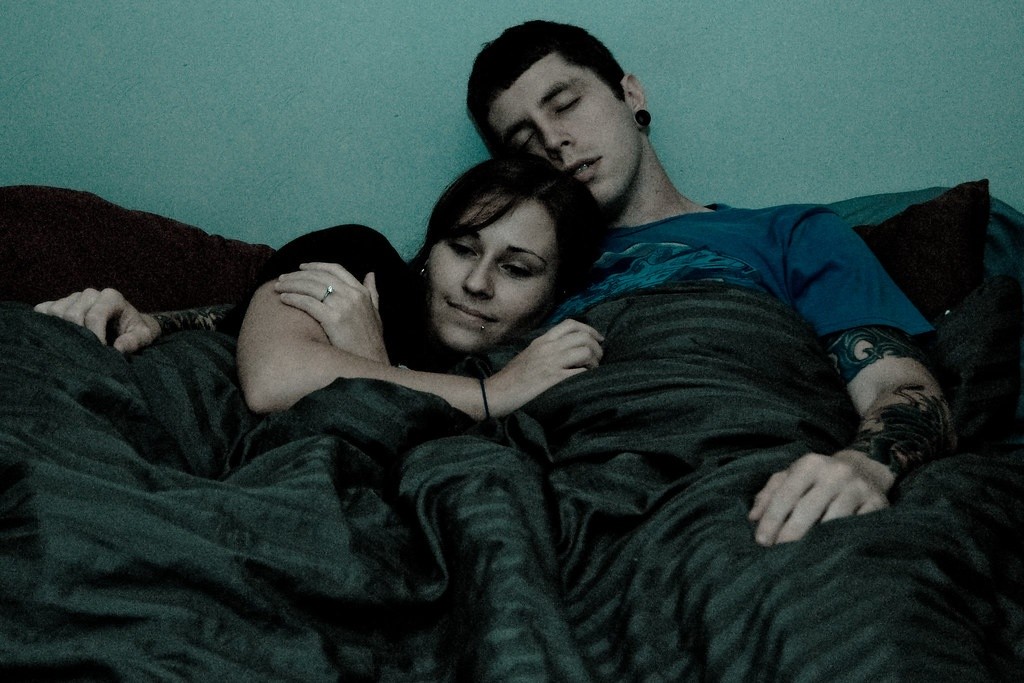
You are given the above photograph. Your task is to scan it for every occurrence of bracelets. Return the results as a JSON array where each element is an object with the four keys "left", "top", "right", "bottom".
[{"left": 480, "top": 379, "right": 490, "bottom": 419}]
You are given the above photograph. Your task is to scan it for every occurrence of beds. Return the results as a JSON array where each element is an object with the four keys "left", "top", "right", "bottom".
[{"left": 0, "top": 186, "right": 1024, "bottom": 683}]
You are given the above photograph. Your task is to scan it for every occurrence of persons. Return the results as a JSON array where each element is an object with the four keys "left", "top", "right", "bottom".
[
  {"left": 38, "top": 21, "right": 960, "bottom": 549},
  {"left": 235, "top": 156, "right": 609, "bottom": 418}
]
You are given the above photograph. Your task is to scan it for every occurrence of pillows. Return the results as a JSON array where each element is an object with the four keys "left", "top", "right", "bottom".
[
  {"left": 0, "top": 184, "right": 273, "bottom": 314},
  {"left": 923, "top": 272, "right": 1024, "bottom": 454},
  {"left": 849, "top": 178, "right": 991, "bottom": 324}
]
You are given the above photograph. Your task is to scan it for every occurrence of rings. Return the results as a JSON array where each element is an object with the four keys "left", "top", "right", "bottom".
[{"left": 320, "top": 286, "right": 333, "bottom": 303}]
